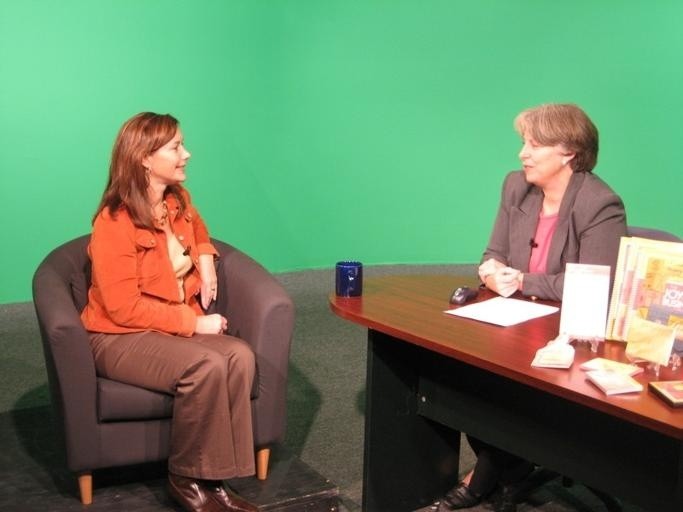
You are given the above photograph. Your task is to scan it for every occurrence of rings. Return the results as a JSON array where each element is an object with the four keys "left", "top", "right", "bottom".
[{"left": 212, "top": 287, "right": 216, "bottom": 291}]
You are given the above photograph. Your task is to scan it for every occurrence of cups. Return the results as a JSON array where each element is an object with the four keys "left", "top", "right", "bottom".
[{"left": 334, "top": 261, "right": 363, "bottom": 296}]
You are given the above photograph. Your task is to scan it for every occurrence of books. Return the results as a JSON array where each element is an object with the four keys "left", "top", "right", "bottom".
[
  {"left": 579, "top": 358, "right": 682, "bottom": 407},
  {"left": 530, "top": 342, "right": 575, "bottom": 368}
]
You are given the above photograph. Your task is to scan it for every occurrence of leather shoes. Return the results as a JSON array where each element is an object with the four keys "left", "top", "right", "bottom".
[
  {"left": 195, "top": 475, "right": 259, "bottom": 512},
  {"left": 441, "top": 469, "right": 522, "bottom": 512},
  {"left": 164, "top": 471, "right": 229, "bottom": 512}
]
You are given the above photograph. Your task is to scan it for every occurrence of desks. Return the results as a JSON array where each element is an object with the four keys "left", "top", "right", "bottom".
[{"left": 326, "top": 275, "right": 683, "bottom": 512}]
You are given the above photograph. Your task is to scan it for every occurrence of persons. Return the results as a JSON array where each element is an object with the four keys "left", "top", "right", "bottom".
[
  {"left": 444, "top": 103, "right": 627, "bottom": 511},
  {"left": 81, "top": 111, "right": 260, "bottom": 512}
]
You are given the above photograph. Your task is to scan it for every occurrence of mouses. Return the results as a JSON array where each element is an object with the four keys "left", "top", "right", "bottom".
[{"left": 449, "top": 286, "right": 478, "bottom": 304}]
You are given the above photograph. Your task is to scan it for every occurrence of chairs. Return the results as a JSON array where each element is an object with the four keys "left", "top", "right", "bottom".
[{"left": 31, "top": 233, "right": 296, "bottom": 506}]
